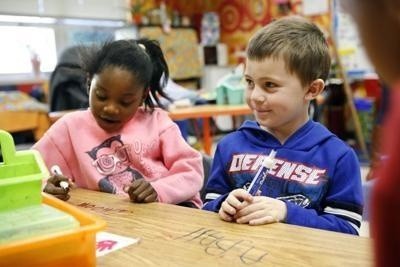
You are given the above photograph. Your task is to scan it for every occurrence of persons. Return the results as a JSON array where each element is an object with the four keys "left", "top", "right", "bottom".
[
  {"left": 31, "top": 30, "right": 205, "bottom": 209},
  {"left": 339, "top": 0, "right": 400, "bottom": 267},
  {"left": 200, "top": 15, "right": 365, "bottom": 235}
]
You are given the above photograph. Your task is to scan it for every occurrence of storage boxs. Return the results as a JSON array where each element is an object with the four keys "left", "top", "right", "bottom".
[{"left": 0, "top": 188, "right": 109, "bottom": 267}]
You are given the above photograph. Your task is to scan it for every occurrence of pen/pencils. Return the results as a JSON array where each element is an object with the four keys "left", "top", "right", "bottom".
[
  {"left": 49, "top": 165, "right": 69, "bottom": 192},
  {"left": 242, "top": 148, "right": 278, "bottom": 207}
]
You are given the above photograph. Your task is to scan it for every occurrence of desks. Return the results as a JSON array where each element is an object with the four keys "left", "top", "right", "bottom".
[
  {"left": 49, "top": 181, "right": 378, "bottom": 267},
  {"left": 164, "top": 103, "right": 258, "bottom": 157}
]
